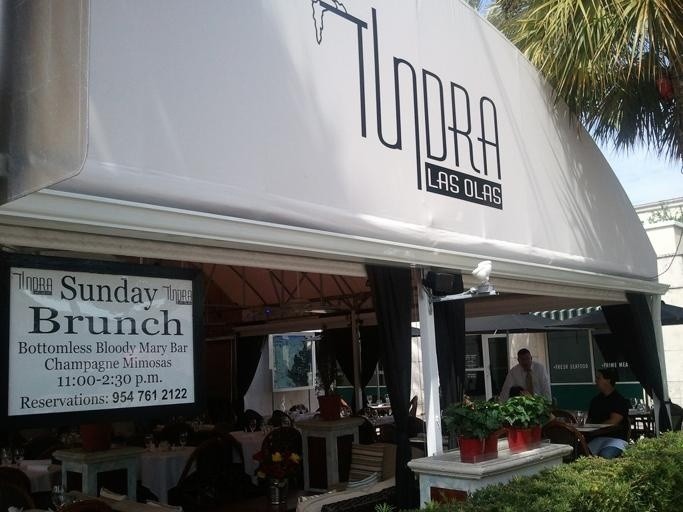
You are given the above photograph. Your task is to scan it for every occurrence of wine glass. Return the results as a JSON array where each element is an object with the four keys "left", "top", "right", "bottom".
[
  {"left": 248, "top": 419, "right": 257, "bottom": 432},
  {"left": 629, "top": 397, "right": 638, "bottom": 411},
  {"left": 179, "top": 438, "right": 187, "bottom": 449},
  {"left": 143, "top": 434, "right": 153, "bottom": 455},
  {"left": 366, "top": 395, "right": 373, "bottom": 405},
  {"left": 13, "top": 447, "right": 24, "bottom": 470}
]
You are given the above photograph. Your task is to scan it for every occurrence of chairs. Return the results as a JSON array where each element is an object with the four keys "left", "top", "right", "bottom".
[{"left": 0, "top": 405, "right": 656, "bottom": 512}]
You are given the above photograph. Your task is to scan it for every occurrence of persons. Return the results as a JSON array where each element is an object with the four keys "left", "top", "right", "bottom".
[
  {"left": 493, "top": 348, "right": 552, "bottom": 406},
  {"left": 508, "top": 386, "right": 530, "bottom": 399},
  {"left": 581, "top": 366, "right": 629, "bottom": 459}
]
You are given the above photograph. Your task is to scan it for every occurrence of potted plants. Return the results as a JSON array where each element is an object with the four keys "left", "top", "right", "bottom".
[
  {"left": 442, "top": 391, "right": 552, "bottom": 464},
  {"left": 314, "top": 325, "right": 342, "bottom": 420}
]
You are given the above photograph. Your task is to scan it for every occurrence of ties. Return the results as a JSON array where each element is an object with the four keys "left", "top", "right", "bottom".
[{"left": 525, "top": 369, "right": 534, "bottom": 395}]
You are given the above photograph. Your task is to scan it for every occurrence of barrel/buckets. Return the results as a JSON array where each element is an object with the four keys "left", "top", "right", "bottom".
[
  {"left": 266, "top": 478, "right": 288, "bottom": 506},
  {"left": 317, "top": 395, "right": 340, "bottom": 421}
]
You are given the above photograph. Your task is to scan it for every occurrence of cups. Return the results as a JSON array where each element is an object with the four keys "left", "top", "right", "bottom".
[{"left": 51, "top": 484, "right": 67, "bottom": 510}]
